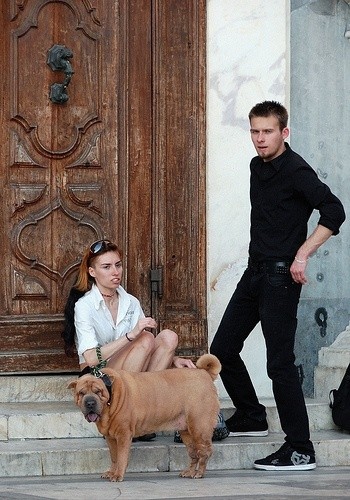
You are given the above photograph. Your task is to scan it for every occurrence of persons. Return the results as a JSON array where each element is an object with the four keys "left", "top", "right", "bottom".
[
  {"left": 73, "top": 239, "right": 198, "bottom": 442},
  {"left": 209, "top": 101, "right": 346, "bottom": 471}
]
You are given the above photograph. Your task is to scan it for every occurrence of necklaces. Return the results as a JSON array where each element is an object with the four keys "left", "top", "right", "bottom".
[{"left": 101, "top": 291, "right": 116, "bottom": 297}]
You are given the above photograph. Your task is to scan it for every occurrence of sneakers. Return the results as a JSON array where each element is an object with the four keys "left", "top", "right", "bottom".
[
  {"left": 254, "top": 439, "right": 317, "bottom": 471},
  {"left": 226, "top": 412, "right": 269, "bottom": 437}
]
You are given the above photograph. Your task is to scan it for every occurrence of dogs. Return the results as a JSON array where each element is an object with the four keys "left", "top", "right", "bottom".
[{"left": 66, "top": 353, "right": 222, "bottom": 483}]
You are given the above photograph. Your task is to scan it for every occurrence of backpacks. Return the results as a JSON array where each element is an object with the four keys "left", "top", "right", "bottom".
[{"left": 329, "top": 363, "right": 350, "bottom": 434}]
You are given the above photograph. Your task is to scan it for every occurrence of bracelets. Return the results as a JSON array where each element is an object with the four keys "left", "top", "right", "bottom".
[
  {"left": 295, "top": 256, "right": 309, "bottom": 264},
  {"left": 125, "top": 333, "right": 133, "bottom": 342}
]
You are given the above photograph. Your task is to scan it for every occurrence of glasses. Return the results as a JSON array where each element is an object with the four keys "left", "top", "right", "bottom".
[{"left": 88, "top": 239, "right": 112, "bottom": 254}]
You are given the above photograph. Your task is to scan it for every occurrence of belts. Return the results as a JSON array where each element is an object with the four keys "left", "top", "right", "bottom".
[{"left": 247, "top": 256, "right": 294, "bottom": 267}]
